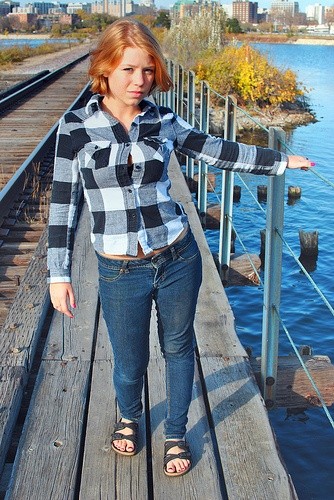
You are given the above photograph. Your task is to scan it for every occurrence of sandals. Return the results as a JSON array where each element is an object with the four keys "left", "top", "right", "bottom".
[
  {"left": 162, "top": 433, "right": 194, "bottom": 477},
  {"left": 109, "top": 416, "right": 139, "bottom": 457}
]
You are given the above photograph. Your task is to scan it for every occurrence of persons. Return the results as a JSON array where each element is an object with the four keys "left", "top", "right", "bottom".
[{"left": 44, "top": 19, "right": 317, "bottom": 478}]
